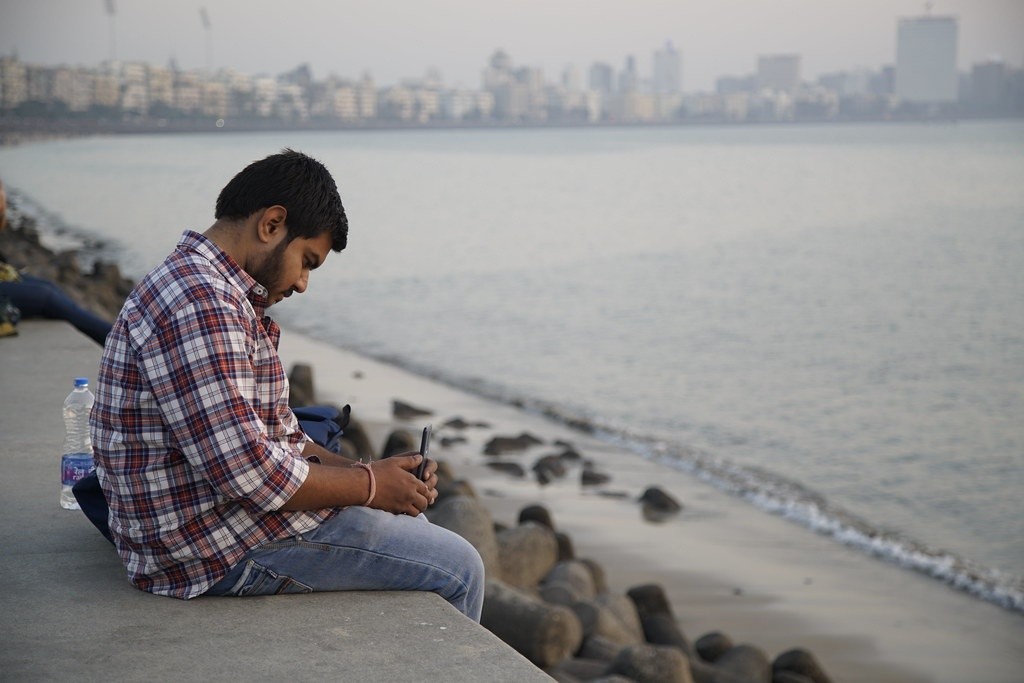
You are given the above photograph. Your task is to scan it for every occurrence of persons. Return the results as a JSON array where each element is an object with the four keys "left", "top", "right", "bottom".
[{"left": 89, "top": 147, "right": 487, "bottom": 626}]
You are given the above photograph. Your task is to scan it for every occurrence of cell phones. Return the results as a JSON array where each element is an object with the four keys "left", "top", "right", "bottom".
[{"left": 416, "top": 424, "right": 433, "bottom": 481}]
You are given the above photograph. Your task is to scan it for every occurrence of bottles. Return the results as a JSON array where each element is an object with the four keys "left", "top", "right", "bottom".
[{"left": 61, "top": 378, "right": 94, "bottom": 511}]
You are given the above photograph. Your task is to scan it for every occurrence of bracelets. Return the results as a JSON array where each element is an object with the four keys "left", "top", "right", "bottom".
[{"left": 349, "top": 462, "right": 376, "bottom": 507}]
